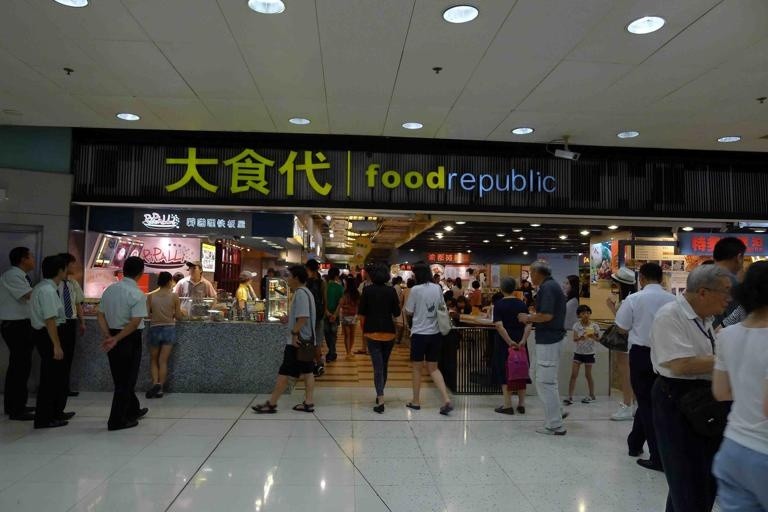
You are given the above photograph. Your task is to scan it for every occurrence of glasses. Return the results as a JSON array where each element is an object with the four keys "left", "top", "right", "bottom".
[{"left": 695, "top": 285, "right": 732, "bottom": 297}]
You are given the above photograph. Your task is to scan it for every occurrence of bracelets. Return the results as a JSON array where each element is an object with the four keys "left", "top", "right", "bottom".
[
  {"left": 527, "top": 314, "right": 531, "bottom": 322},
  {"left": 290, "top": 329, "right": 299, "bottom": 336}
]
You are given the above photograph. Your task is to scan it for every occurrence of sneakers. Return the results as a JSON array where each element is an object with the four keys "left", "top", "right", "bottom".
[
  {"left": 155, "top": 391, "right": 164, "bottom": 398},
  {"left": 536, "top": 427, "right": 566, "bottom": 436},
  {"left": 144, "top": 383, "right": 161, "bottom": 399},
  {"left": 563, "top": 399, "right": 576, "bottom": 405},
  {"left": 617, "top": 401, "right": 636, "bottom": 417},
  {"left": 610, "top": 402, "right": 633, "bottom": 421},
  {"left": 580, "top": 396, "right": 595, "bottom": 402}
]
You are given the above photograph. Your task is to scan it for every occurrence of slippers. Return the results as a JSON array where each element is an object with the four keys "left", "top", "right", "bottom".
[
  {"left": 353, "top": 350, "right": 366, "bottom": 355},
  {"left": 406, "top": 401, "right": 420, "bottom": 411},
  {"left": 439, "top": 403, "right": 453, "bottom": 415}
]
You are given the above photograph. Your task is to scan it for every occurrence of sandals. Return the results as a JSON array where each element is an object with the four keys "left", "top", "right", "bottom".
[
  {"left": 292, "top": 401, "right": 315, "bottom": 413},
  {"left": 252, "top": 400, "right": 277, "bottom": 414}
]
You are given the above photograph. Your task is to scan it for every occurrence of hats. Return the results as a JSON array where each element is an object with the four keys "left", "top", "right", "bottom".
[
  {"left": 287, "top": 265, "right": 308, "bottom": 282},
  {"left": 185, "top": 260, "right": 202, "bottom": 268},
  {"left": 610, "top": 266, "right": 636, "bottom": 285},
  {"left": 317, "top": 363, "right": 325, "bottom": 376},
  {"left": 239, "top": 269, "right": 257, "bottom": 281}
]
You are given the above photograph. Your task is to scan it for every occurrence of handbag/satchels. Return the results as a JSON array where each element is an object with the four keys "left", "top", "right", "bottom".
[
  {"left": 296, "top": 339, "right": 315, "bottom": 362},
  {"left": 598, "top": 324, "right": 627, "bottom": 353},
  {"left": 436, "top": 302, "right": 451, "bottom": 337},
  {"left": 506, "top": 345, "right": 530, "bottom": 382},
  {"left": 701, "top": 400, "right": 733, "bottom": 457},
  {"left": 393, "top": 310, "right": 407, "bottom": 344}
]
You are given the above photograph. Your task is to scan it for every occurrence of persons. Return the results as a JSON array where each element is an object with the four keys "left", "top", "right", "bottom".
[
  {"left": 446, "top": 277, "right": 453, "bottom": 288},
  {"left": 236, "top": 270, "right": 257, "bottom": 301},
  {"left": 580, "top": 276, "right": 590, "bottom": 298},
  {"left": 0, "top": 246, "right": 37, "bottom": 420},
  {"left": 305, "top": 259, "right": 325, "bottom": 367},
  {"left": 356, "top": 261, "right": 401, "bottom": 412},
  {"left": 517, "top": 258, "right": 570, "bottom": 435},
  {"left": 444, "top": 290, "right": 457, "bottom": 304},
  {"left": 443, "top": 293, "right": 452, "bottom": 305},
  {"left": 399, "top": 278, "right": 416, "bottom": 329},
  {"left": 440, "top": 274, "right": 446, "bottom": 287},
  {"left": 404, "top": 260, "right": 453, "bottom": 414},
  {"left": 146, "top": 272, "right": 183, "bottom": 399},
  {"left": 58, "top": 252, "right": 87, "bottom": 396},
  {"left": 172, "top": 261, "right": 217, "bottom": 298},
  {"left": 434, "top": 274, "right": 443, "bottom": 293},
  {"left": 29, "top": 255, "right": 75, "bottom": 429},
  {"left": 392, "top": 276, "right": 402, "bottom": 302},
  {"left": 261, "top": 268, "right": 275, "bottom": 300},
  {"left": 715, "top": 303, "right": 749, "bottom": 328},
  {"left": 562, "top": 304, "right": 602, "bottom": 405},
  {"left": 356, "top": 273, "right": 363, "bottom": 288},
  {"left": 713, "top": 237, "right": 747, "bottom": 328},
  {"left": 337, "top": 271, "right": 345, "bottom": 287},
  {"left": 493, "top": 276, "right": 533, "bottom": 414},
  {"left": 451, "top": 277, "right": 464, "bottom": 300},
  {"left": 614, "top": 263, "right": 677, "bottom": 472},
  {"left": 323, "top": 267, "right": 344, "bottom": 360},
  {"left": 649, "top": 264, "right": 737, "bottom": 512},
  {"left": 347, "top": 273, "right": 353, "bottom": 279},
  {"left": 468, "top": 280, "right": 483, "bottom": 307},
  {"left": 564, "top": 275, "right": 580, "bottom": 331},
  {"left": 339, "top": 277, "right": 361, "bottom": 360},
  {"left": 455, "top": 297, "right": 471, "bottom": 314},
  {"left": 712, "top": 260, "right": 768, "bottom": 512},
  {"left": 606, "top": 267, "right": 637, "bottom": 421},
  {"left": 252, "top": 265, "right": 317, "bottom": 414},
  {"left": 358, "top": 270, "right": 373, "bottom": 295},
  {"left": 521, "top": 280, "right": 532, "bottom": 307},
  {"left": 322, "top": 273, "right": 328, "bottom": 281},
  {"left": 98, "top": 256, "right": 149, "bottom": 429}
]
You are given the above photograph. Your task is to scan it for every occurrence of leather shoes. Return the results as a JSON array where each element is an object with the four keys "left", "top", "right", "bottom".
[
  {"left": 22, "top": 406, "right": 36, "bottom": 412},
  {"left": 373, "top": 404, "right": 384, "bottom": 413},
  {"left": 636, "top": 459, "right": 664, "bottom": 473},
  {"left": 494, "top": 404, "right": 513, "bottom": 415},
  {"left": 9, "top": 413, "right": 34, "bottom": 421},
  {"left": 62, "top": 412, "right": 75, "bottom": 419},
  {"left": 33, "top": 418, "right": 68, "bottom": 429},
  {"left": 107, "top": 419, "right": 138, "bottom": 431},
  {"left": 67, "top": 392, "right": 79, "bottom": 396},
  {"left": 130, "top": 408, "right": 148, "bottom": 419},
  {"left": 516, "top": 406, "right": 525, "bottom": 414}
]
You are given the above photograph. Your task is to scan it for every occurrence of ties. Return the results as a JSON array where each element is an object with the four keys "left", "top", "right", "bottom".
[
  {"left": 56, "top": 289, "right": 60, "bottom": 298},
  {"left": 25, "top": 274, "right": 33, "bottom": 288},
  {"left": 62, "top": 279, "right": 73, "bottom": 318}
]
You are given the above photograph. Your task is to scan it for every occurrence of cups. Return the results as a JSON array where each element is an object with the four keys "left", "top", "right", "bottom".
[{"left": 258, "top": 312, "right": 265, "bottom": 321}]
